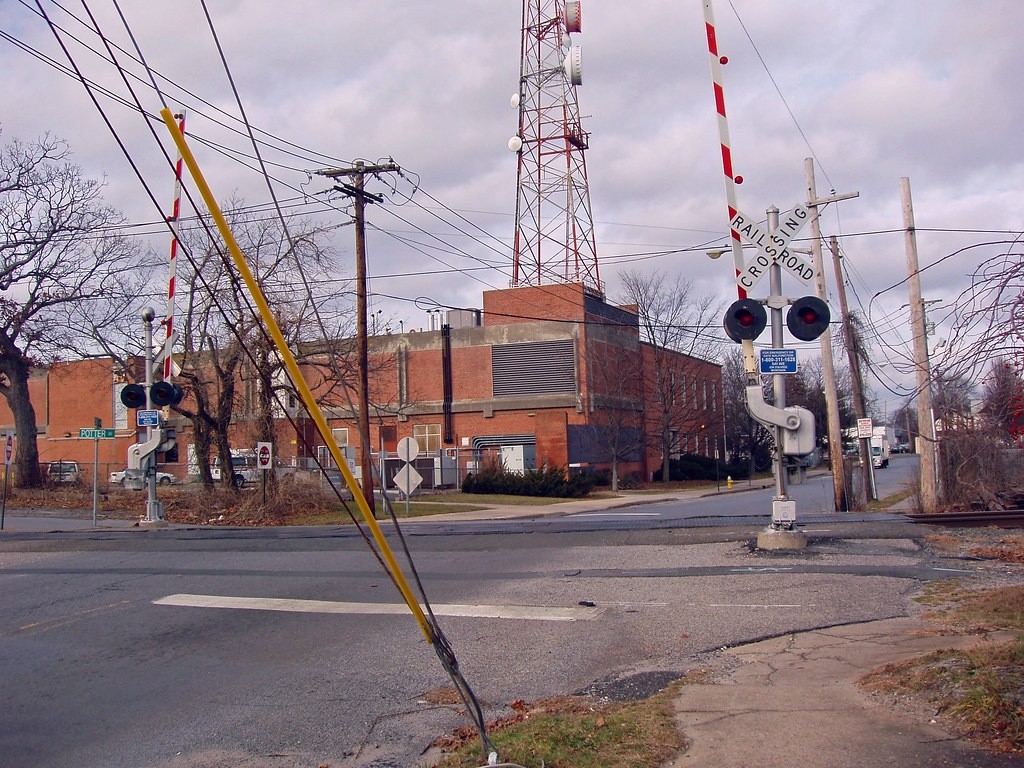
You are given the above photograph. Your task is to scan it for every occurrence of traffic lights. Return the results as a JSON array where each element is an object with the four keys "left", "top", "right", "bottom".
[
  {"left": 120, "top": 384, "right": 146, "bottom": 409},
  {"left": 725, "top": 298, "right": 768, "bottom": 340},
  {"left": 150, "top": 380, "right": 174, "bottom": 406},
  {"left": 786, "top": 296, "right": 831, "bottom": 339}
]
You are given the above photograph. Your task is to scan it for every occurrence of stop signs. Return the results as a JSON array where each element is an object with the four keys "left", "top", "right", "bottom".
[
  {"left": 4, "top": 430, "right": 14, "bottom": 465},
  {"left": 256, "top": 442, "right": 273, "bottom": 470}
]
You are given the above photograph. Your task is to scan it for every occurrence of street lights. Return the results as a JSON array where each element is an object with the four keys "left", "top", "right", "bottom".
[{"left": 705, "top": 245, "right": 849, "bottom": 512}]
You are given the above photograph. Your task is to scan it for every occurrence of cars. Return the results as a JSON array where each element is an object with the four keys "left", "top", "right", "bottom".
[
  {"left": 887, "top": 440, "right": 910, "bottom": 454},
  {"left": 109, "top": 466, "right": 177, "bottom": 489}
]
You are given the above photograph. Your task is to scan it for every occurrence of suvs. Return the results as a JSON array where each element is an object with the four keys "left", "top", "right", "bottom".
[
  {"left": 46, "top": 460, "right": 84, "bottom": 487},
  {"left": 211, "top": 446, "right": 295, "bottom": 488}
]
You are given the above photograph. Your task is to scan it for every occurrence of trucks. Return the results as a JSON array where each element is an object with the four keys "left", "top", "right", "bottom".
[{"left": 858, "top": 435, "right": 891, "bottom": 469}]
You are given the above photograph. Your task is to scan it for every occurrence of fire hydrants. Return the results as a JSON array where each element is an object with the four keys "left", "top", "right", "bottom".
[{"left": 727, "top": 475, "right": 733, "bottom": 490}]
